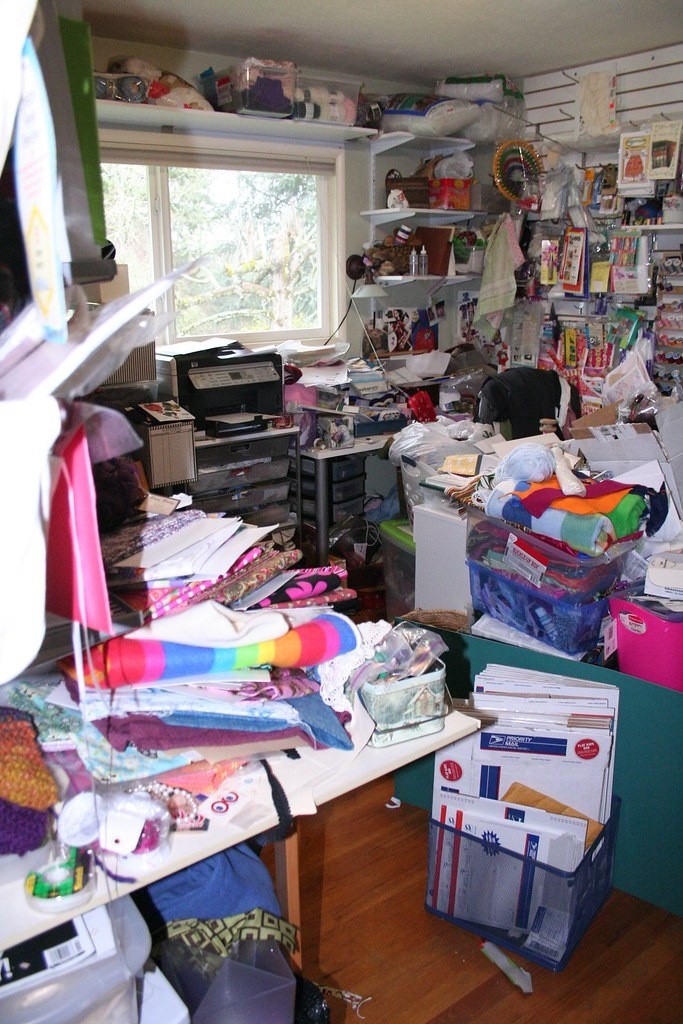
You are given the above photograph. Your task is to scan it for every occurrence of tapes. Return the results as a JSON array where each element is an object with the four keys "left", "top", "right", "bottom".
[{"left": 25, "top": 858, "right": 95, "bottom": 916}]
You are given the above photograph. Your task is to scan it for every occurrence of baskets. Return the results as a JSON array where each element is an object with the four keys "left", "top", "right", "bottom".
[{"left": 425, "top": 794, "right": 622, "bottom": 971}]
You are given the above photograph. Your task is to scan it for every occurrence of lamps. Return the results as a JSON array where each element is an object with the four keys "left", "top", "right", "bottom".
[{"left": 346, "top": 254, "right": 387, "bottom": 299}]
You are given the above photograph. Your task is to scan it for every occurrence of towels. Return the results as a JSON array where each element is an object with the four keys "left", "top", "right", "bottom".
[
  {"left": 468, "top": 212, "right": 526, "bottom": 343},
  {"left": 437, "top": 442, "right": 671, "bottom": 603},
  {"left": 0, "top": 83, "right": 76, "bottom": 346},
  {"left": 145, "top": 839, "right": 284, "bottom": 923},
  {"left": 60, "top": 596, "right": 361, "bottom": 755}
]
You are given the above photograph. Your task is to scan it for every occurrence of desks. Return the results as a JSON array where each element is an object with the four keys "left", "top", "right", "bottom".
[{"left": 0, "top": 705, "right": 497, "bottom": 1024}]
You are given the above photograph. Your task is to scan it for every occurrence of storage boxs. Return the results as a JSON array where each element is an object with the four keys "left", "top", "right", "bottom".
[
  {"left": 385, "top": 177, "right": 430, "bottom": 208},
  {"left": 83, "top": 264, "right": 131, "bottom": 305},
  {"left": 130, "top": 419, "right": 198, "bottom": 488},
  {"left": 453, "top": 244, "right": 485, "bottom": 275},
  {"left": 295, "top": 75, "right": 364, "bottom": 126},
  {"left": 424, "top": 797, "right": 622, "bottom": 972},
  {"left": 358, "top": 637, "right": 446, "bottom": 747},
  {"left": 199, "top": 66, "right": 296, "bottom": 118},
  {"left": 0, "top": 896, "right": 151, "bottom": 1024},
  {"left": 465, "top": 505, "right": 643, "bottom": 605},
  {"left": 430, "top": 178, "right": 471, "bottom": 211},
  {"left": 603, "top": 597, "right": 683, "bottom": 693},
  {"left": 466, "top": 560, "right": 608, "bottom": 657}
]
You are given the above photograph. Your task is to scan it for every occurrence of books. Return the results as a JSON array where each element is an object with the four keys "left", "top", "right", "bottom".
[{"left": 424, "top": 664, "right": 620, "bottom": 948}]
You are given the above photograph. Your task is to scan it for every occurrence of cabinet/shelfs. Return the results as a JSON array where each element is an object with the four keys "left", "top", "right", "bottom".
[
  {"left": 368, "top": 131, "right": 485, "bottom": 389},
  {"left": 285, "top": 431, "right": 402, "bottom": 574},
  {"left": 187, "top": 425, "right": 302, "bottom": 559}
]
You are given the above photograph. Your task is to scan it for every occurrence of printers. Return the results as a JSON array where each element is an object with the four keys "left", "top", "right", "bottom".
[{"left": 154, "top": 341, "right": 284, "bottom": 438}]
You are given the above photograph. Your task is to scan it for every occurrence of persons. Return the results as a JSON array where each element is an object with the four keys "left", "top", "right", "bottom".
[
  {"left": 473, "top": 368, "right": 582, "bottom": 441},
  {"left": 391, "top": 309, "right": 412, "bottom": 352}
]
